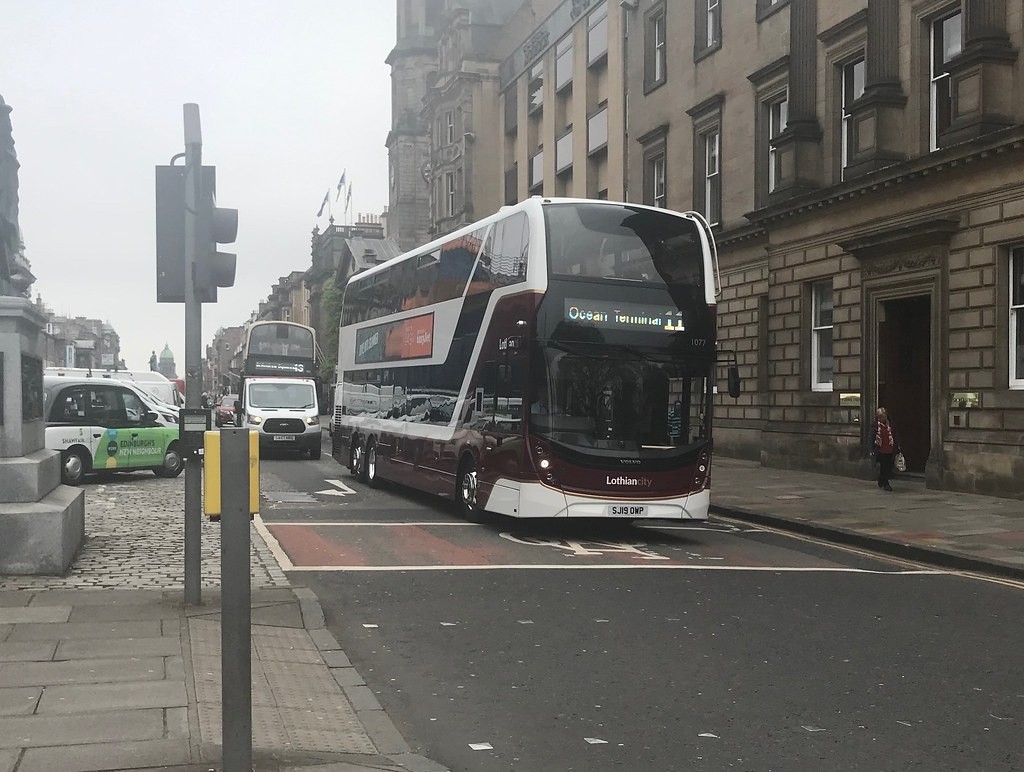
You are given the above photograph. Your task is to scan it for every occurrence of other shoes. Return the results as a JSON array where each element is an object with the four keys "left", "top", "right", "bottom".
[{"left": 878, "top": 481, "right": 893, "bottom": 491}]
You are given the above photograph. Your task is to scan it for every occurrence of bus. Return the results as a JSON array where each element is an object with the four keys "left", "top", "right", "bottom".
[
  {"left": 226, "top": 321, "right": 320, "bottom": 397},
  {"left": 332, "top": 195, "right": 743, "bottom": 526}
]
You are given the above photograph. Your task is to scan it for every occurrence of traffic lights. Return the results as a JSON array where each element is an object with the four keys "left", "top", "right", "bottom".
[{"left": 201, "top": 164, "right": 239, "bottom": 304}]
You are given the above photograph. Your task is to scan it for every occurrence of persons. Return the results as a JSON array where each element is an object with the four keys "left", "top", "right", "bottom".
[
  {"left": 871, "top": 407, "right": 899, "bottom": 491},
  {"left": 667, "top": 400, "right": 692, "bottom": 445},
  {"left": 148, "top": 350, "right": 157, "bottom": 371},
  {"left": 532, "top": 381, "right": 563, "bottom": 415}
]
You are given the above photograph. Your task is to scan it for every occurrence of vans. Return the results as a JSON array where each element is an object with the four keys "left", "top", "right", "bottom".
[
  {"left": 45, "top": 366, "right": 187, "bottom": 424},
  {"left": 241, "top": 376, "right": 322, "bottom": 459}
]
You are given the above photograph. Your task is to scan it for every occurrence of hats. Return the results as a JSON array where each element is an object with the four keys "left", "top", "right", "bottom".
[{"left": 535, "top": 347, "right": 568, "bottom": 370}]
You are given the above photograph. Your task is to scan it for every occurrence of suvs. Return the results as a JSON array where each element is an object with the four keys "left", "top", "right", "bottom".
[
  {"left": 39, "top": 374, "right": 186, "bottom": 487},
  {"left": 214, "top": 393, "right": 241, "bottom": 428}
]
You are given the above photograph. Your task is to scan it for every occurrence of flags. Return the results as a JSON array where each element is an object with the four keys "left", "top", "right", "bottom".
[
  {"left": 337, "top": 173, "right": 344, "bottom": 200},
  {"left": 346, "top": 185, "right": 352, "bottom": 213},
  {"left": 317, "top": 192, "right": 329, "bottom": 217}
]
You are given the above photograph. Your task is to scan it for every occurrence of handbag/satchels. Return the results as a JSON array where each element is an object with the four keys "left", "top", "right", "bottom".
[{"left": 894, "top": 449, "right": 906, "bottom": 471}]
